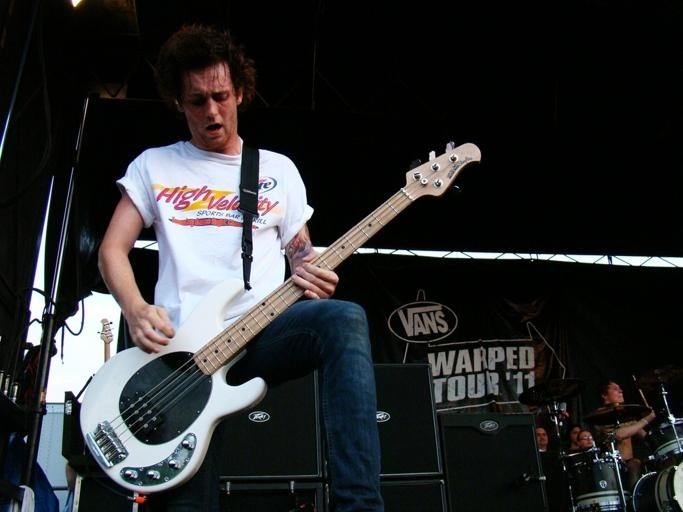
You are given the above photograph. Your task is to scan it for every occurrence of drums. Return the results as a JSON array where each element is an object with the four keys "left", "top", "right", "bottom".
[
  {"left": 644, "top": 418, "right": 683, "bottom": 463},
  {"left": 567, "top": 459, "right": 628, "bottom": 511},
  {"left": 633, "top": 463, "right": 682, "bottom": 510}
]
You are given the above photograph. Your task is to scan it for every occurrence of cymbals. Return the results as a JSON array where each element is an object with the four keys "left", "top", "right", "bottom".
[
  {"left": 636, "top": 364, "right": 682, "bottom": 391},
  {"left": 582, "top": 405, "right": 651, "bottom": 424},
  {"left": 518, "top": 378, "right": 582, "bottom": 405}
]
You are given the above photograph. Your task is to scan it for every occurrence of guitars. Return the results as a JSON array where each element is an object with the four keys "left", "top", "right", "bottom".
[{"left": 79, "top": 143, "right": 481, "bottom": 492}]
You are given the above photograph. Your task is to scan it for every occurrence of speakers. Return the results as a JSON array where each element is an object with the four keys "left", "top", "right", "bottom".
[
  {"left": 213, "top": 368, "right": 322, "bottom": 480},
  {"left": 324, "top": 479, "right": 448, "bottom": 512},
  {"left": 324, "top": 363, "right": 443, "bottom": 478},
  {"left": 218, "top": 480, "right": 324, "bottom": 512},
  {"left": 440, "top": 411, "right": 548, "bottom": 512}
]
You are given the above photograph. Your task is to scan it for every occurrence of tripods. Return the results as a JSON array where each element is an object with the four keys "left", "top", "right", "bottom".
[
  {"left": 545, "top": 398, "right": 576, "bottom": 512},
  {"left": 602, "top": 430, "right": 627, "bottom": 512},
  {"left": 1, "top": 310, "right": 57, "bottom": 512},
  {"left": 659, "top": 389, "right": 683, "bottom": 453}
]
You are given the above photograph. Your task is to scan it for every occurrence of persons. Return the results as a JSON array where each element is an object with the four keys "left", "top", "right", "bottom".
[
  {"left": 534, "top": 381, "right": 656, "bottom": 512},
  {"left": 96, "top": 23, "right": 385, "bottom": 512}
]
